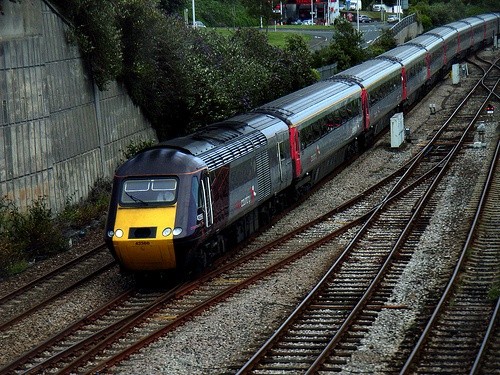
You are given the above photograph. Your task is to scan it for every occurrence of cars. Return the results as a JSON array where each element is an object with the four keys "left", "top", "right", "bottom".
[
  {"left": 386, "top": 16, "right": 399, "bottom": 23},
  {"left": 373, "top": 5, "right": 387, "bottom": 13},
  {"left": 188, "top": 21, "right": 206, "bottom": 28},
  {"left": 359, "top": 15, "right": 371, "bottom": 23}
]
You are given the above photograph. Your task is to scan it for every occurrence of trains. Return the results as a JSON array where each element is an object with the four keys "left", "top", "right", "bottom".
[{"left": 103, "top": 12, "right": 500, "bottom": 274}]
